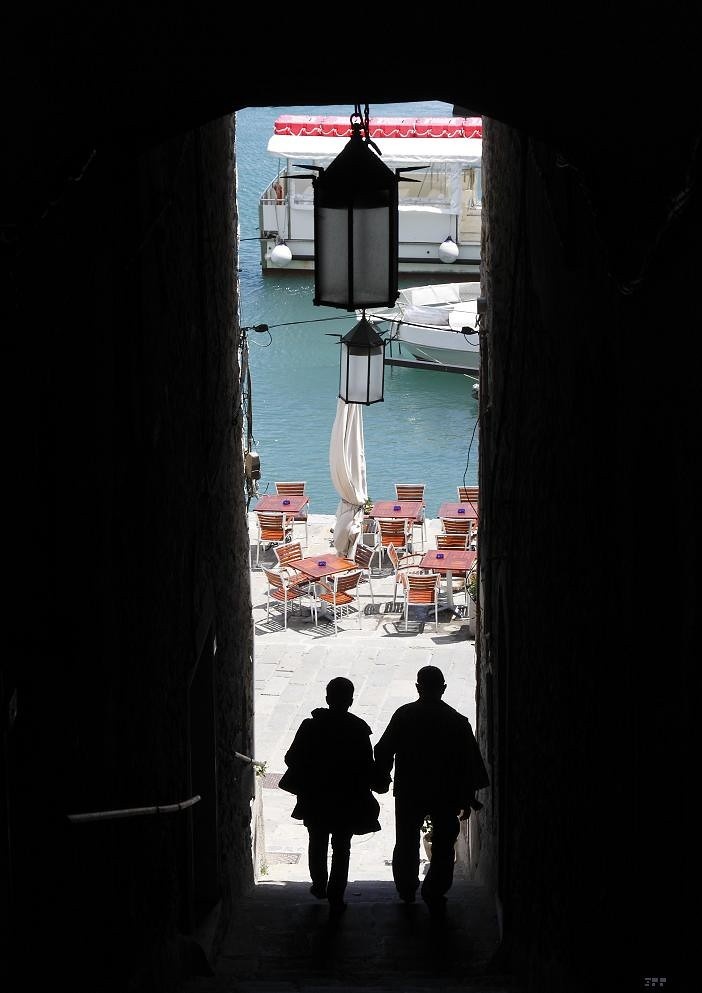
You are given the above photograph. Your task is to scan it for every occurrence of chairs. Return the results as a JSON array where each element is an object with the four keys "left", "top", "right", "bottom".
[{"left": 254, "top": 482, "right": 477, "bottom": 633}]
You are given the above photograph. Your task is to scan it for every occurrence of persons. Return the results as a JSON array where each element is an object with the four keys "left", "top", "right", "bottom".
[
  {"left": 278, "top": 677, "right": 381, "bottom": 902},
  {"left": 373, "top": 666, "right": 491, "bottom": 903}
]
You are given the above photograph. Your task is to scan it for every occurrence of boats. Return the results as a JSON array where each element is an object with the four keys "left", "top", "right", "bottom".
[
  {"left": 366, "top": 281, "right": 481, "bottom": 368},
  {"left": 258, "top": 114, "right": 483, "bottom": 276}
]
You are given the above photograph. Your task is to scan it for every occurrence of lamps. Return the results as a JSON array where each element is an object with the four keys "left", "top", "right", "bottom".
[
  {"left": 338, "top": 309, "right": 384, "bottom": 405},
  {"left": 309, "top": 101, "right": 398, "bottom": 312}
]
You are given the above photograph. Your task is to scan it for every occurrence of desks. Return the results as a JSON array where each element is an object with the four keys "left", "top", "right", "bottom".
[
  {"left": 368, "top": 501, "right": 423, "bottom": 563},
  {"left": 436, "top": 503, "right": 478, "bottom": 525},
  {"left": 420, "top": 550, "right": 476, "bottom": 615},
  {"left": 253, "top": 495, "right": 310, "bottom": 547},
  {"left": 287, "top": 553, "right": 359, "bottom": 622}
]
